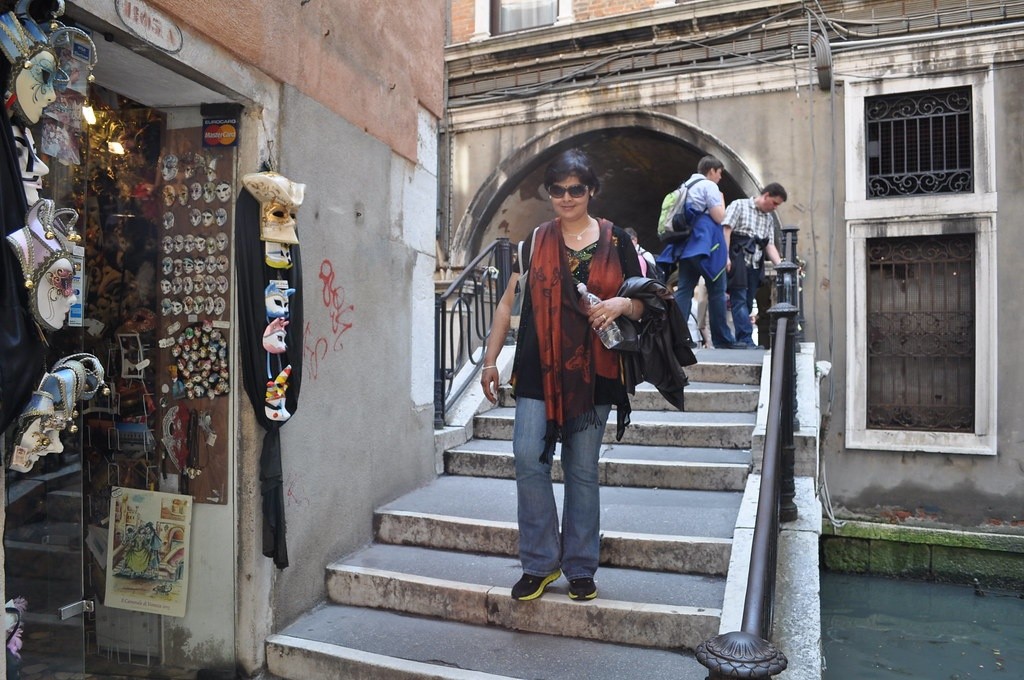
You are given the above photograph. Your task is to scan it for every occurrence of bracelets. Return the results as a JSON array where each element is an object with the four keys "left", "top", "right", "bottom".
[
  {"left": 480, "top": 364, "right": 498, "bottom": 371},
  {"left": 625, "top": 296, "right": 635, "bottom": 316}
]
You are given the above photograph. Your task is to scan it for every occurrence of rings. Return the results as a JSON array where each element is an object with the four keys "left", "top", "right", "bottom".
[{"left": 599, "top": 314, "right": 607, "bottom": 321}]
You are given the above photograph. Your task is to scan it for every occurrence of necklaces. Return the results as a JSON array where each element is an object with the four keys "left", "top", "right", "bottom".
[{"left": 562, "top": 223, "right": 593, "bottom": 242}]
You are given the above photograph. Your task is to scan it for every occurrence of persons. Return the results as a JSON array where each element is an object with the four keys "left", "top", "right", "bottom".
[
  {"left": 622, "top": 155, "right": 787, "bottom": 349},
  {"left": 480, "top": 149, "right": 648, "bottom": 602}
]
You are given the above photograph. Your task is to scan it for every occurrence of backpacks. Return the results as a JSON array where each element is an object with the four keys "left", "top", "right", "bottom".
[{"left": 657, "top": 178, "right": 709, "bottom": 244}]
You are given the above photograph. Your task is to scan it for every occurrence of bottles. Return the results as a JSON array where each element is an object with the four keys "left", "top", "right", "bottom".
[{"left": 576, "top": 283, "right": 624, "bottom": 349}]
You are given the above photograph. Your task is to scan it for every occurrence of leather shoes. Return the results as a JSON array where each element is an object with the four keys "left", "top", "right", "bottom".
[
  {"left": 716, "top": 342, "right": 747, "bottom": 349},
  {"left": 689, "top": 341, "right": 697, "bottom": 347}
]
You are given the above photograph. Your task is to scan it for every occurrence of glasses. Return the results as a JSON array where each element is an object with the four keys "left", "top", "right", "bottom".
[{"left": 546, "top": 184, "right": 589, "bottom": 197}]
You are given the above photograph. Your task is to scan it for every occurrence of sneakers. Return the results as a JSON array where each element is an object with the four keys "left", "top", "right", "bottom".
[
  {"left": 511, "top": 570, "right": 561, "bottom": 600},
  {"left": 569, "top": 578, "right": 598, "bottom": 600}
]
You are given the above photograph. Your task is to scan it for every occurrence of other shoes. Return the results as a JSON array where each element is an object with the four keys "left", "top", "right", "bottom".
[{"left": 749, "top": 343, "right": 764, "bottom": 349}]
